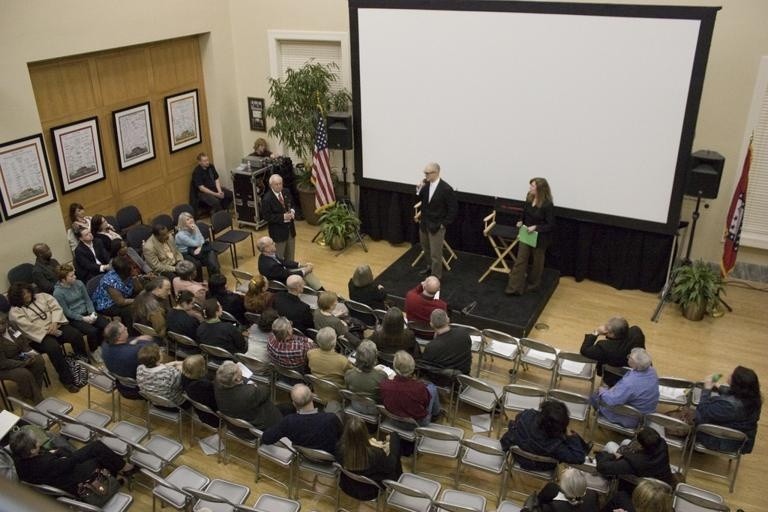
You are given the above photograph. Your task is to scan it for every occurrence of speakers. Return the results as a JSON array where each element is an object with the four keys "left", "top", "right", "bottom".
[
  {"left": 328, "top": 115, "right": 351, "bottom": 149},
  {"left": 681, "top": 149, "right": 725, "bottom": 199}
]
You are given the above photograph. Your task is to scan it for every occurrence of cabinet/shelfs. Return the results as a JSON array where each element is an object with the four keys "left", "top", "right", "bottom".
[{"left": 228, "top": 162, "right": 273, "bottom": 231}]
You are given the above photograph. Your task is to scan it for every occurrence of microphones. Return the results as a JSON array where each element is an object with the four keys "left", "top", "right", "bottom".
[
  {"left": 416, "top": 179, "right": 427, "bottom": 196},
  {"left": 287, "top": 202, "right": 291, "bottom": 215}
]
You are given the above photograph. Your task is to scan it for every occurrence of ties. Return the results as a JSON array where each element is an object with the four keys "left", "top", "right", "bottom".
[{"left": 278, "top": 193, "right": 285, "bottom": 209}]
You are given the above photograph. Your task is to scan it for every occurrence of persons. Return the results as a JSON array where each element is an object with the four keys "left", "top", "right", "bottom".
[
  {"left": 589, "top": 347, "right": 660, "bottom": 427},
  {"left": 498, "top": 401, "right": 593, "bottom": 474},
  {"left": 581, "top": 316, "right": 645, "bottom": 388},
  {"left": 604, "top": 477, "right": 676, "bottom": 511},
  {"left": 596, "top": 425, "right": 671, "bottom": 485},
  {"left": 502, "top": 176, "right": 559, "bottom": 296},
  {"left": 521, "top": 463, "right": 603, "bottom": 511},
  {"left": 688, "top": 365, "right": 761, "bottom": 453}
]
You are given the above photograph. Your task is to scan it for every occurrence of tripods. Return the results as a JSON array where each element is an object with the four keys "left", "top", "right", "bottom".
[
  {"left": 311, "top": 149, "right": 368, "bottom": 253},
  {"left": 651, "top": 196, "right": 733, "bottom": 321}
]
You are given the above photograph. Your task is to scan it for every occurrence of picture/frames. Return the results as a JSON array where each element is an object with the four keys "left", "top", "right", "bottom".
[
  {"left": 110, "top": 99, "right": 156, "bottom": 173},
  {"left": 247, "top": 96, "right": 267, "bottom": 133},
  {"left": 50, "top": 115, "right": 106, "bottom": 196},
  {"left": 0, "top": 132, "right": 61, "bottom": 222},
  {"left": 163, "top": 86, "right": 204, "bottom": 155}
]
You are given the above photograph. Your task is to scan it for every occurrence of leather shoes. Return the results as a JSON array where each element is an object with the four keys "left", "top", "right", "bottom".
[
  {"left": 417, "top": 267, "right": 430, "bottom": 275},
  {"left": 525, "top": 285, "right": 542, "bottom": 293},
  {"left": 506, "top": 288, "right": 524, "bottom": 295}
]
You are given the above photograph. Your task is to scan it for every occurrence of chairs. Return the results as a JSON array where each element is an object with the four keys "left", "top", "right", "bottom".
[
  {"left": 432, "top": 329, "right": 596, "bottom": 512},
  {"left": 559, "top": 363, "right": 748, "bottom": 511},
  {"left": 295, "top": 293, "right": 479, "bottom": 511},
  {"left": 1, "top": 204, "right": 318, "bottom": 511}
]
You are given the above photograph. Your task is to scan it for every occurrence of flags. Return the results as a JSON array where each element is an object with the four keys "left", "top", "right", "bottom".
[
  {"left": 310, "top": 95, "right": 336, "bottom": 214},
  {"left": 721, "top": 138, "right": 752, "bottom": 277}
]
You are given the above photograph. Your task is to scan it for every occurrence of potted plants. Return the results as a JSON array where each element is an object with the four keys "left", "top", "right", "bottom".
[{"left": 266, "top": 59, "right": 352, "bottom": 223}]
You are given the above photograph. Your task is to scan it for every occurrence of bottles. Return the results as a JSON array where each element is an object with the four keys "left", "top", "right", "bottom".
[
  {"left": 710, "top": 374, "right": 722, "bottom": 383},
  {"left": 247, "top": 161, "right": 252, "bottom": 172}
]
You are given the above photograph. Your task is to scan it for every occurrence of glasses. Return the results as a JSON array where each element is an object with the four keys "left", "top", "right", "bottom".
[{"left": 424, "top": 170, "right": 436, "bottom": 175}]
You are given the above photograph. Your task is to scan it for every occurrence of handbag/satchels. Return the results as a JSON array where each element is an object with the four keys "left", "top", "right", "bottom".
[{"left": 76, "top": 468, "right": 122, "bottom": 510}]
